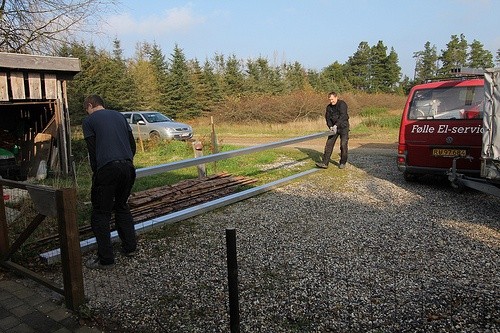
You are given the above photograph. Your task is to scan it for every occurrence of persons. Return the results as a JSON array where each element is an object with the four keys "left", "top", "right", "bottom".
[
  {"left": 315, "top": 92, "right": 350, "bottom": 169},
  {"left": 82, "top": 95, "right": 137, "bottom": 269},
  {"left": 0, "top": 126, "right": 22, "bottom": 181}
]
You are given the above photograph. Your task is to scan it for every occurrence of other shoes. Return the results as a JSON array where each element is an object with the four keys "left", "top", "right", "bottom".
[
  {"left": 86, "top": 259, "right": 115, "bottom": 270},
  {"left": 315, "top": 162, "right": 328, "bottom": 168},
  {"left": 126, "top": 246, "right": 137, "bottom": 256},
  {"left": 339, "top": 164, "right": 345, "bottom": 169}
]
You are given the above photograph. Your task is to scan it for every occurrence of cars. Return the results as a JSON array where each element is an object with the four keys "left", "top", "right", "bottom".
[{"left": 119, "top": 111, "right": 194, "bottom": 141}]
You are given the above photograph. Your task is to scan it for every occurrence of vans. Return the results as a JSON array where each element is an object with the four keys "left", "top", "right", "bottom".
[{"left": 396, "top": 65, "right": 500, "bottom": 191}]
